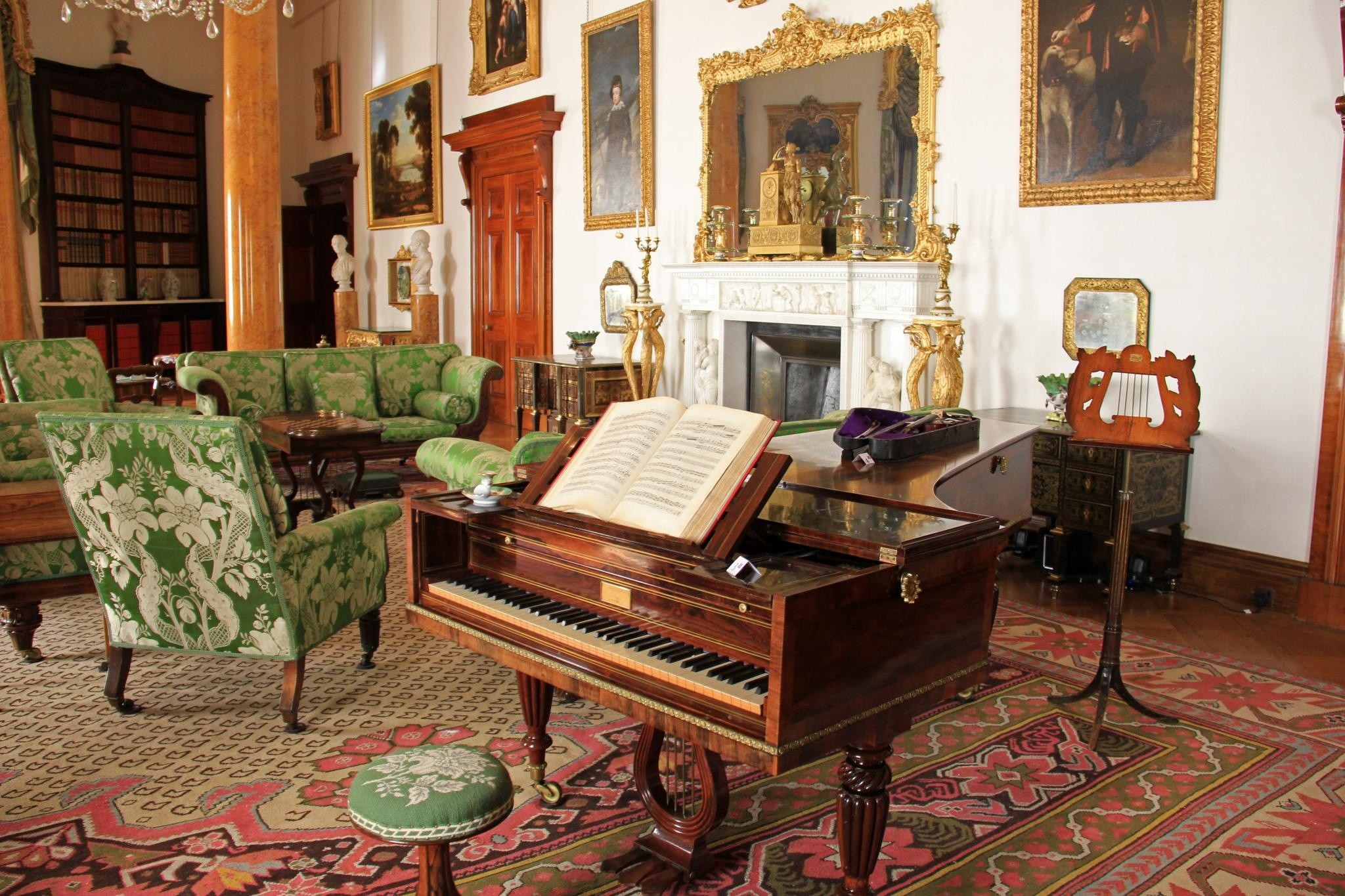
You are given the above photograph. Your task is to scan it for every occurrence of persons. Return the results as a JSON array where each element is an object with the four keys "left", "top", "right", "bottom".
[
  {"left": 409, "top": 229, "right": 435, "bottom": 295},
  {"left": 694, "top": 337, "right": 718, "bottom": 405},
  {"left": 332, "top": 235, "right": 356, "bottom": 292},
  {"left": 773, "top": 142, "right": 805, "bottom": 224},
  {"left": 806, "top": 151, "right": 852, "bottom": 227},
  {"left": 864, "top": 356, "right": 901, "bottom": 412}
]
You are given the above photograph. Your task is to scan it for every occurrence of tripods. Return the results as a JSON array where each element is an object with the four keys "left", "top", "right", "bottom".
[{"left": 1047, "top": 345, "right": 1202, "bottom": 752}]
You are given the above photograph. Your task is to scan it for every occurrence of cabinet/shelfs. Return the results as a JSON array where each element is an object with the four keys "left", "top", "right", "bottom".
[
  {"left": 27, "top": 58, "right": 213, "bottom": 301},
  {"left": 974, "top": 407, "right": 1195, "bottom": 596}
]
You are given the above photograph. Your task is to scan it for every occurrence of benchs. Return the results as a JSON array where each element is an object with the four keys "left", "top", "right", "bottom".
[
  {"left": 175, "top": 342, "right": 503, "bottom": 482},
  {"left": 415, "top": 407, "right": 975, "bottom": 495}
]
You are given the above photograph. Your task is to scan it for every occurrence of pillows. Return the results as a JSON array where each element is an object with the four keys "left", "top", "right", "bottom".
[
  {"left": 414, "top": 389, "right": 473, "bottom": 425},
  {"left": 303, "top": 368, "right": 380, "bottom": 422}
]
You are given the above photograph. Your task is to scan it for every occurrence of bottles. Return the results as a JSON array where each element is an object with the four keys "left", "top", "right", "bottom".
[
  {"left": 161, "top": 269, "right": 180, "bottom": 300},
  {"left": 97, "top": 270, "right": 118, "bottom": 302}
]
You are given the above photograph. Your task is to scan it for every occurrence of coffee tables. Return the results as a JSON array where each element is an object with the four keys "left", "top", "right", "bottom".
[{"left": 256, "top": 410, "right": 384, "bottom": 529}]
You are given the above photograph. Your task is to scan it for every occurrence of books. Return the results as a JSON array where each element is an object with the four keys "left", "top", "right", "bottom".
[
  {"left": 52, "top": 90, "right": 199, "bottom": 301},
  {"left": 538, "top": 397, "right": 782, "bottom": 548}
]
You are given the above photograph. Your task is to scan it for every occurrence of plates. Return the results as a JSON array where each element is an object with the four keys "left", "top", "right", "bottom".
[{"left": 461, "top": 486, "right": 512, "bottom": 504}]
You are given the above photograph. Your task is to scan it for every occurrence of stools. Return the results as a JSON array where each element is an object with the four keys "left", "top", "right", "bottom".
[{"left": 348, "top": 743, "right": 514, "bottom": 896}]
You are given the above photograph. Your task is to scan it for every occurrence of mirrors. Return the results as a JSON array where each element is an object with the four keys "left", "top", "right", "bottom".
[
  {"left": 1062, "top": 277, "right": 1151, "bottom": 361},
  {"left": 693, "top": 0, "right": 946, "bottom": 262}
]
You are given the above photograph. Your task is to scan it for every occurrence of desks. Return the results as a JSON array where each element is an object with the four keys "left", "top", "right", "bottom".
[
  {"left": 40, "top": 302, "right": 226, "bottom": 397},
  {"left": 510, "top": 354, "right": 658, "bottom": 443}
]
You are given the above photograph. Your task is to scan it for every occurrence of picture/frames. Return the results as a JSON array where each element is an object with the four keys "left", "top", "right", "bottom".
[
  {"left": 467, "top": 0, "right": 541, "bottom": 97},
  {"left": 580, "top": 1, "right": 654, "bottom": 231},
  {"left": 362, "top": 64, "right": 443, "bottom": 230},
  {"left": 312, "top": 61, "right": 340, "bottom": 140},
  {"left": 1021, "top": 0, "right": 1224, "bottom": 208}
]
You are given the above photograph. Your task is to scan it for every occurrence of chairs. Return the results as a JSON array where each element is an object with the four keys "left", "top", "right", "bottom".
[
  {"left": 0, "top": 336, "right": 204, "bottom": 667},
  {"left": 36, "top": 407, "right": 403, "bottom": 735}
]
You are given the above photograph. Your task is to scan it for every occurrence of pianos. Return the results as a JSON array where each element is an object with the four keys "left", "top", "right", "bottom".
[{"left": 402, "top": 410, "right": 1040, "bottom": 896}]
